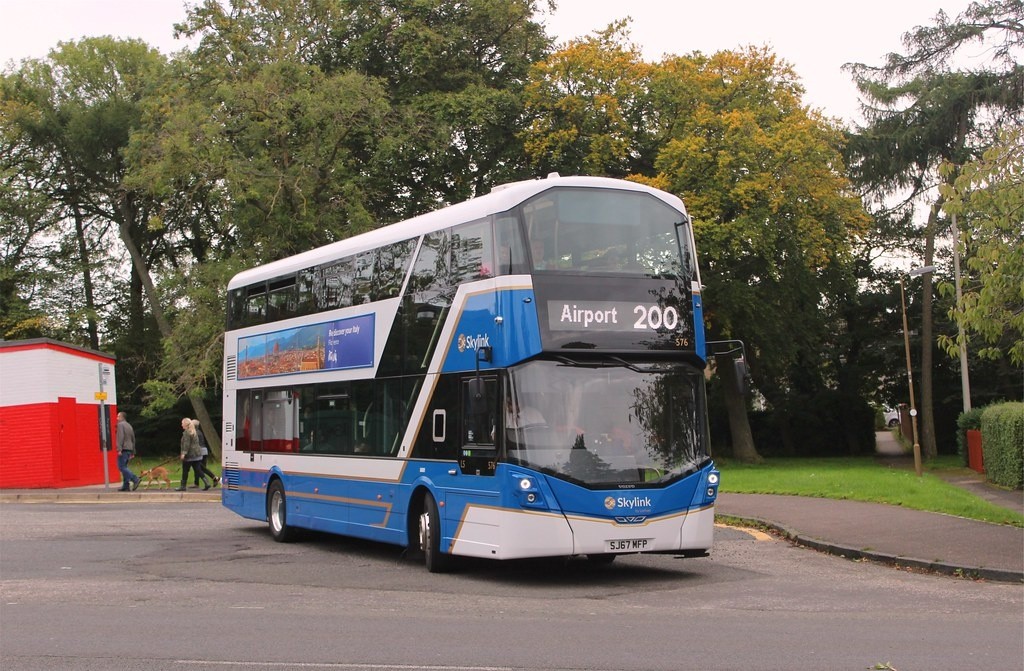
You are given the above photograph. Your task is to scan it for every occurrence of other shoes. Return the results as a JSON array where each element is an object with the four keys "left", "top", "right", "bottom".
[
  {"left": 213, "top": 477, "right": 220, "bottom": 487},
  {"left": 189, "top": 484, "right": 199, "bottom": 488},
  {"left": 132, "top": 478, "right": 141, "bottom": 491},
  {"left": 118, "top": 486, "right": 130, "bottom": 491}
]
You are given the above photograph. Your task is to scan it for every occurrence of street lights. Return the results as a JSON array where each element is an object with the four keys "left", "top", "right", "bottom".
[{"left": 900, "top": 265, "right": 937, "bottom": 477}]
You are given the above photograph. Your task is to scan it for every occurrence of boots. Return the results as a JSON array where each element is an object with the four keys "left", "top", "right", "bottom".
[
  {"left": 175, "top": 480, "right": 186, "bottom": 491},
  {"left": 202, "top": 476, "right": 211, "bottom": 491}
]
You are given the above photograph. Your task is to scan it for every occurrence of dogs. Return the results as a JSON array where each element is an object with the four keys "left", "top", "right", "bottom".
[{"left": 140, "top": 466, "right": 181, "bottom": 491}]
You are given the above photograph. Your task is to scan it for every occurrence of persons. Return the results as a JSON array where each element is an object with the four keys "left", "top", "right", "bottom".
[
  {"left": 116, "top": 412, "right": 142, "bottom": 491},
  {"left": 601, "top": 247, "right": 621, "bottom": 269},
  {"left": 174, "top": 418, "right": 211, "bottom": 491},
  {"left": 555, "top": 413, "right": 585, "bottom": 437},
  {"left": 479, "top": 245, "right": 510, "bottom": 277},
  {"left": 243, "top": 400, "right": 376, "bottom": 456},
  {"left": 491, "top": 394, "right": 547, "bottom": 440},
  {"left": 527, "top": 238, "right": 547, "bottom": 270},
  {"left": 189, "top": 419, "right": 220, "bottom": 488},
  {"left": 612, "top": 420, "right": 645, "bottom": 484}
]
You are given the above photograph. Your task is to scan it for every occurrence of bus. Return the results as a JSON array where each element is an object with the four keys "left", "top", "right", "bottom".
[{"left": 221, "top": 174, "right": 749, "bottom": 573}]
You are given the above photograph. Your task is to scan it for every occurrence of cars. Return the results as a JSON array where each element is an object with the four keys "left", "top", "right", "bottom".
[{"left": 868, "top": 401, "right": 901, "bottom": 429}]
